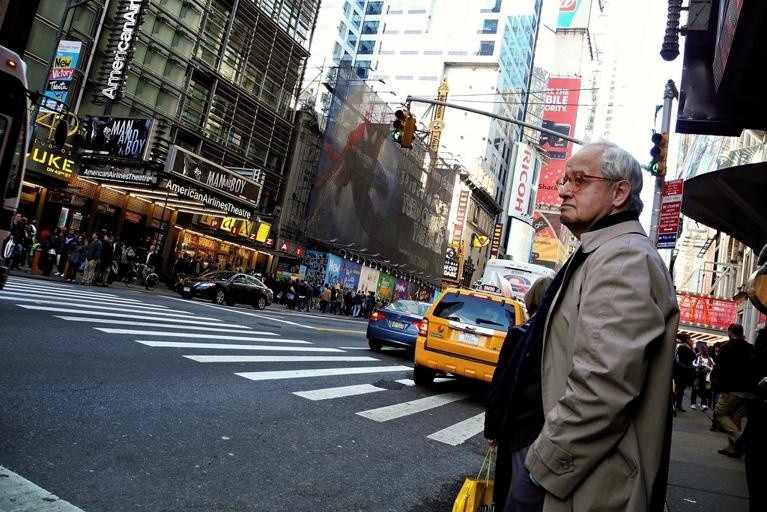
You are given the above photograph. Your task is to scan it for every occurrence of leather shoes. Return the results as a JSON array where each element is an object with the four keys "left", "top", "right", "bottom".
[{"left": 719, "top": 448, "right": 738, "bottom": 457}]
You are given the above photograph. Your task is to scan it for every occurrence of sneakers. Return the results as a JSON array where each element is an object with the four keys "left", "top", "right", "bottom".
[{"left": 691, "top": 404, "right": 708, "bottom": 412}]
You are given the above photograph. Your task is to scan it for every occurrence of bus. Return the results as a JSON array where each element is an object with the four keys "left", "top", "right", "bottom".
[
  {"left": 481, "top": 259, "right": 556, "bottom": 302},
  {"left": 0, "top": 45, "right": 70, "bottom": 291}
]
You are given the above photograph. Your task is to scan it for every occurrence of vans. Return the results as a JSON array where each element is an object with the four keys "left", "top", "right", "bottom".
[{"left": 413, "top": 270, "right": 530, "bottom": 405}]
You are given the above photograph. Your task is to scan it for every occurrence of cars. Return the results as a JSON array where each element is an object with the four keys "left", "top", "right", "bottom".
[
  {"left": 175, "top": 270, "right": 273, "bottom": 310},
  {"left": 366, "top": 299, "right": 433, "bottom": 362}
]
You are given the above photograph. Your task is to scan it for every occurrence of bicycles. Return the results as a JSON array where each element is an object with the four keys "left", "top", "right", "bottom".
[{"left": 123, "top": 262, "right": 159, "bottom": 291}]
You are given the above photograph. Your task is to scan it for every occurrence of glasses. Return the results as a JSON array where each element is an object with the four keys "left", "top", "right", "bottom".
[{"left": 556, "top": 173, "right": 615, "bottom": 192}]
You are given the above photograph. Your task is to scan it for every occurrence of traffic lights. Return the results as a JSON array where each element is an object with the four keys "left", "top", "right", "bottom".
[
  {"left": 406, "top": 117, "right": 417, "bottom": 149},
  {"left": 649, "top": 132, "right": 668, "bottom": 177},
  {"left": 392, "top": 110, "right": 409, "bottom": 143}
]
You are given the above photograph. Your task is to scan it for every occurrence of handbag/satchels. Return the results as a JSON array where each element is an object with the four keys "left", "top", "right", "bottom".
[{"left": 452, "top": 448, "right": 495, "bottom": 512}]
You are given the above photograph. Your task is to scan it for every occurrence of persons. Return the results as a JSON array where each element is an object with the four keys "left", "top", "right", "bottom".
[
  {"left": 484, "top": 277, "right": 556, "bottom": 512},
  {"left": 692, "top": 344, "right": 716, "bottom": 410},
  {"left": 673, "top": 333, "right": 696, "bottom": 410},
  {"left": 334, "top": 105, "right": 390, "bottom": 232},
  {"left": 712, "top": 324, "right": 756, "bottom": 459},
  {"left": 712, "top": 343, "right": 720, "bottom": 363},
  {"left": 525, "top": 143, "right": 681, "bottom": 512},
  {"left": 747, "top": 326, "right": 767, "bottom": 512}
]
